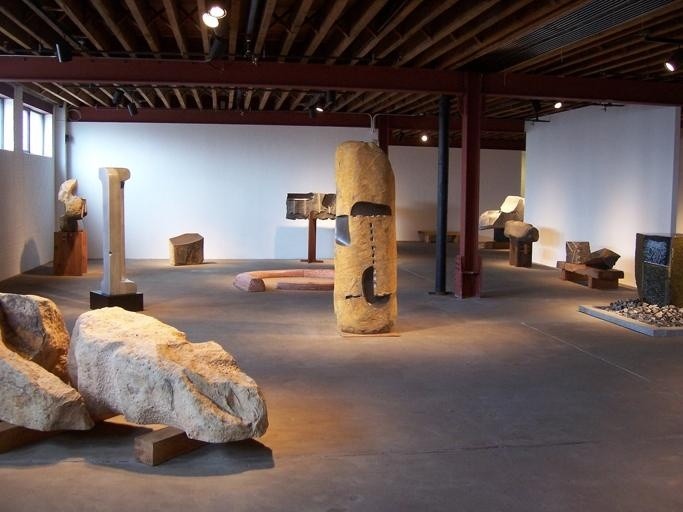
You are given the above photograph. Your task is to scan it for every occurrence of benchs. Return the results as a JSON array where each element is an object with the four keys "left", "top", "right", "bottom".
[{"left": 418, "top": 228, "right": 460, "bottom": 244}]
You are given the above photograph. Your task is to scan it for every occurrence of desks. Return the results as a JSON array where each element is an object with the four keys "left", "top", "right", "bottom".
[{"left": 556, "top": 260, "right": 625, "bottom": 288}]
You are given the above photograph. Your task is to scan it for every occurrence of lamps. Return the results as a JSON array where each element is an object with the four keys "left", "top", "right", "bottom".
[
  {"left": 111, "top": 86, "right": 138, "bottom": 116},
  {"left": 53, "top": 34, "right": 77, "bottom": 64},
  {"left": 313, "top": 96, "right": 328, "bottom": 114}
]
